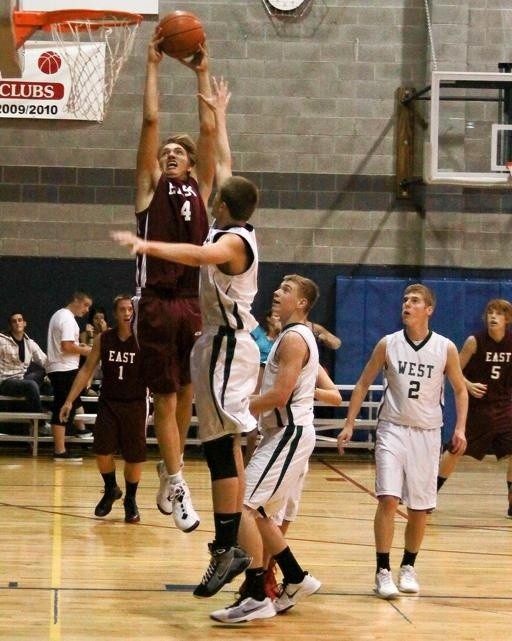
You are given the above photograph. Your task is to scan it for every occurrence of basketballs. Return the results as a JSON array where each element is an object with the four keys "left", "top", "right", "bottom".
[{"left": 158, "top": 11, "right": 204, "bottom": 59}]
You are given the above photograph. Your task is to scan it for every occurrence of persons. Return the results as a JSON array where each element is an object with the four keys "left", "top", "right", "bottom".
[
  {"left": 434, "top": 299, "right": 510, "bottom": 512},
  {"left": 110, "top": 20, "right": 259, "bottom": 536},
  {"left": 203, "top": 270, "right": 322, "bottom": 623},
  {"left": 336, "top": 283, "right": 470, "bottom": 599},
  {"left": 0, "top": 296, "right": 147, "bottom": 522},
  {"left": 237, "top": 464, "right": 311, "bottom": 600},
  {"left": 109, "top": 74, "right": 256, "bottom": 597},
  {"left": 250, "top": 303, "right": 341, "bottom": 405}
]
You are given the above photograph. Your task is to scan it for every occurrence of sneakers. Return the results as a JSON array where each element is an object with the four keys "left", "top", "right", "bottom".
[
  {"left": 376, "top": 568, "right": 399, "bottom": 599},
  {"left": 399, "top": 565, "right": 419, "bottom": 592},
  {"left": 76, "top": 429, "right": 93, "bottom": 437},
  {"left": 52, "top": 450, "right": 83, "bottom": 462},
  {"left": 94, "top": 484, "right": 141, "bottom": 523},
  {"left": 190, "top": 540, "right": 322, "bottom": 624},
  {"left": 156, "top": 457, "right": 201, "bottom": 534}
]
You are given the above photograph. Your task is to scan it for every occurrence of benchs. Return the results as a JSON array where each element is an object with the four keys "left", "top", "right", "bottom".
[{"left": 1, "top": 383, "right": 388, "bottom": 454}]
